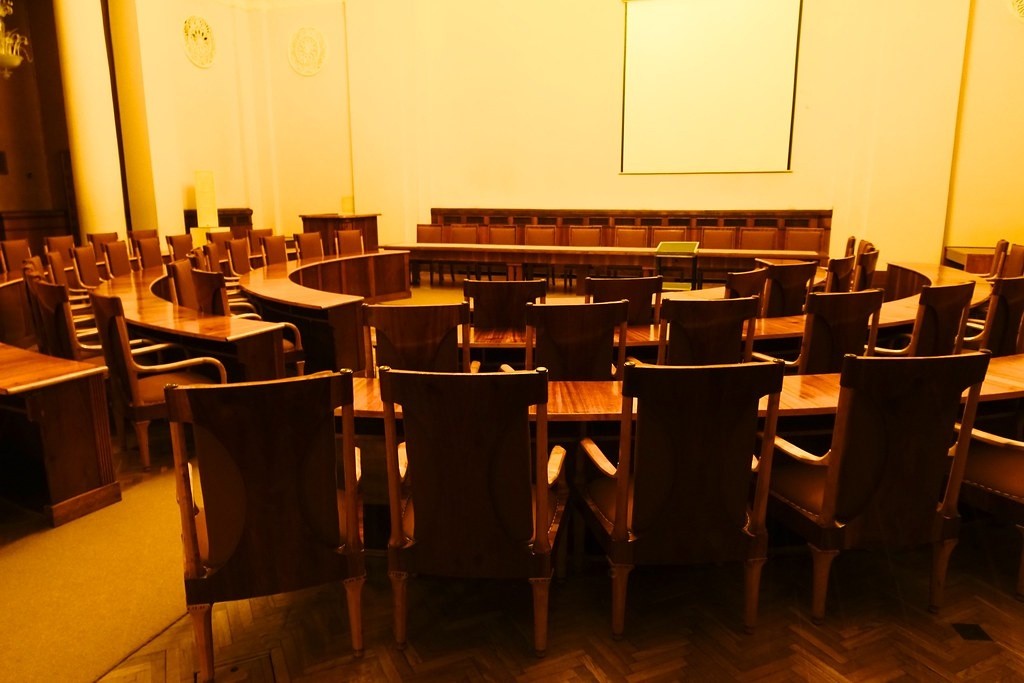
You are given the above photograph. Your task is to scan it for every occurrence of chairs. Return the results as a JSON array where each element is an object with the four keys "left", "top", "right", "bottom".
[{"left": 2, "top": 197, "right": 1024, "bottom": 683}]
